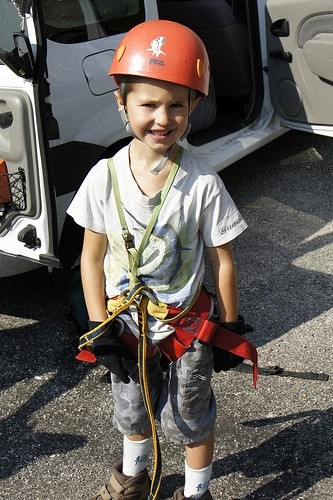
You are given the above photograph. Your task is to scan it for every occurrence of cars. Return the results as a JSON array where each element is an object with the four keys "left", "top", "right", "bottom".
[{"left": 0, "top": 0, "right": 333, "bottom": 273}]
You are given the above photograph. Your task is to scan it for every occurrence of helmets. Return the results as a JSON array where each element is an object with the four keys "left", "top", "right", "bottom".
[{"left": 106, "top": 20, "right": 210, "bottom": 97}]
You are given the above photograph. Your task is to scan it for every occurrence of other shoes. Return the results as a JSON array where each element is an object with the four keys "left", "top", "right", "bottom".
[
  {"left": 169, "top": 487, "right": 214, "bottom": 500},
  {"left": 89, "top": 461, "right": 151, "bottom": 500}
]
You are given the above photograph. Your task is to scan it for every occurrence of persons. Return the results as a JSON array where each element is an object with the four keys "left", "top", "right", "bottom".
[{"left": 66, "top": 20, "right": 248, "bottom": 499}]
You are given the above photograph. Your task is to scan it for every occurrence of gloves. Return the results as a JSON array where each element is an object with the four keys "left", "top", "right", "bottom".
[
  {"left": 212, "top": 315, "right": 249, "bottom": 374},
  {"left": 87, "top": 319, "right": 138, "bottom": 384}
]
